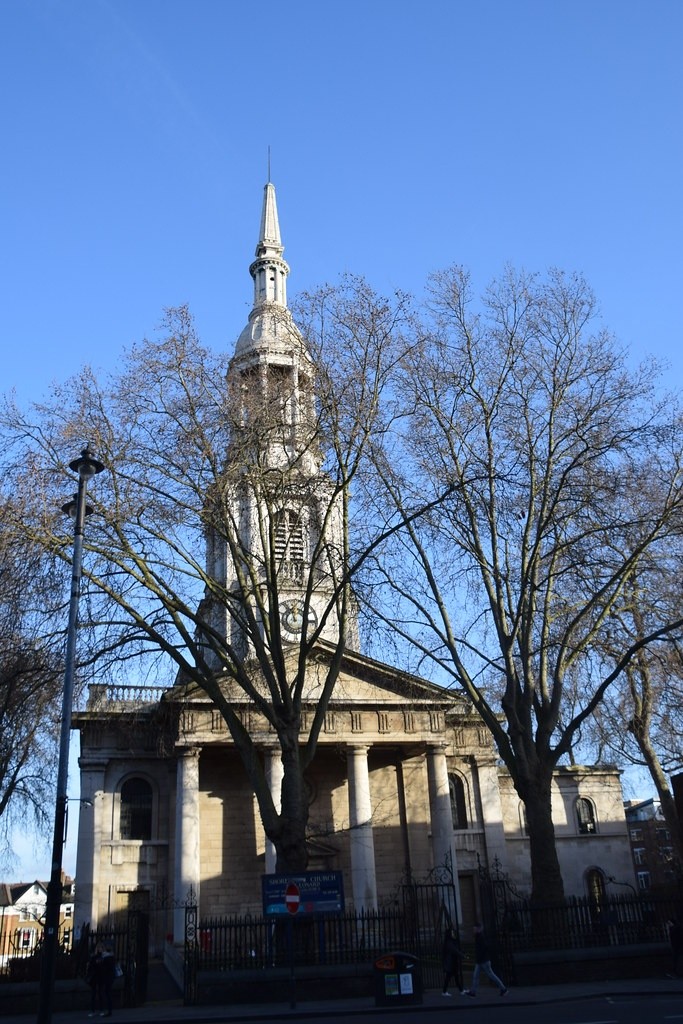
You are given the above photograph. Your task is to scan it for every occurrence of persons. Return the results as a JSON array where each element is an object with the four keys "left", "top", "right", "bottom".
[
  {"left": 441, "top": 928, "right": 470, "bottom": 998},
  {"left": 463, "top": 921, "right": 508, "bottom": 998},
  {"left": 86, "top": 943, "right": 120, "bottom": 1017},
  {"left": 665, "top": 918, "right": 683, "bottom": 978}
]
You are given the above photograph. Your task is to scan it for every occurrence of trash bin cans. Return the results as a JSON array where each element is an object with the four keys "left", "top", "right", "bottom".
[{"left": 373, "top": 951, "right": 424, "bottom": 1007}]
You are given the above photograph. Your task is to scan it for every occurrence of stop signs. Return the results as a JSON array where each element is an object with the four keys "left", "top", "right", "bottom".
[{"left": 284, "top": 882, "right": 301, "bottom": 915}]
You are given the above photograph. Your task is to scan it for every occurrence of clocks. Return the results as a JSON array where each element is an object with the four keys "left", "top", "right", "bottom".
[{"left": 272, "top": 599, "right": 319, "bottom": 645}]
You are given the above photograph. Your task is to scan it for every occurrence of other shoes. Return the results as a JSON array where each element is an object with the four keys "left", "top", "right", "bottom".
[
  {"left": 500, "top": 990, "right": 509, "bottom": 998},
  {"left": 460, "top": 989, "right": 470, "bottom": 995},
  {"left": 441, "top": 992, "right": 452, "bottom": 997},
  {"left": 464, "top": 991, "right": 476, "bottom": 998}
]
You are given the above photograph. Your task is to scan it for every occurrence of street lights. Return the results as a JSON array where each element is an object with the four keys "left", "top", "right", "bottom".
[{"left": 32, "top": 443, "right": 107, "bottom": 1024}]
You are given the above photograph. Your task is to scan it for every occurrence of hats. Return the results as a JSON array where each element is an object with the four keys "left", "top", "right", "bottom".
[{"left": 471, "top": 921, "right": 482, "bottom": 929}]
